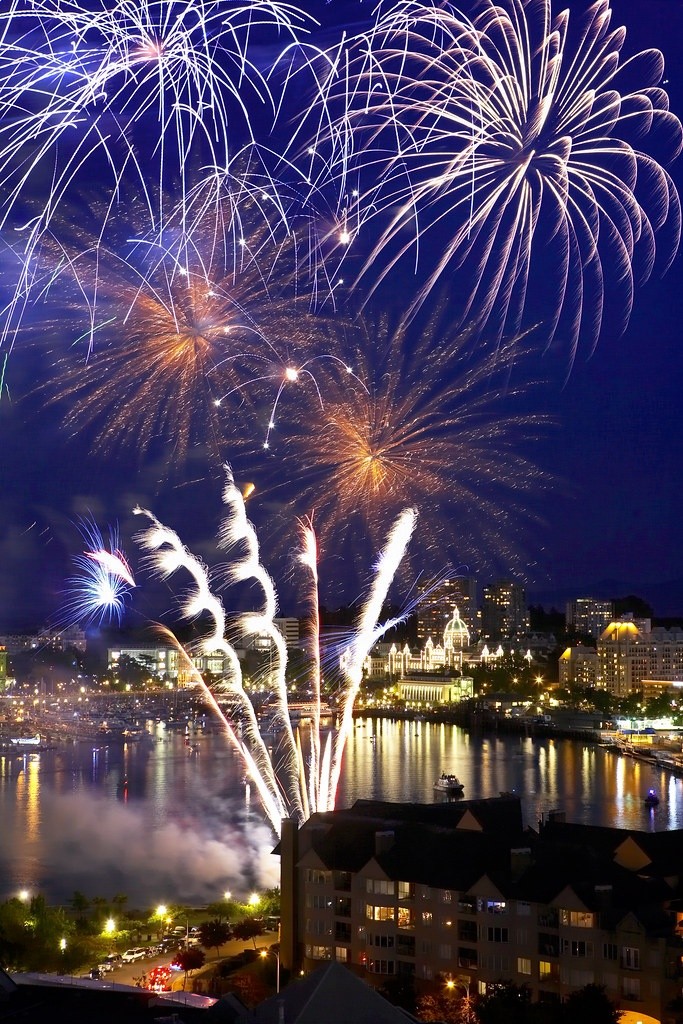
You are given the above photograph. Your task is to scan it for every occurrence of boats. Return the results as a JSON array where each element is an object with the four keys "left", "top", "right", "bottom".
[
  {"left": 10, "top": 733, "right": 41, "bottom": 745},
  {"left": 432, "top": 769, "right": 464, "bottom": 794}
]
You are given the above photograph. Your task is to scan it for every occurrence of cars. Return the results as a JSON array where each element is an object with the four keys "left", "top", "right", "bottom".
[{"left": 156, "top": 938, "right": 182, "bottom": 954}]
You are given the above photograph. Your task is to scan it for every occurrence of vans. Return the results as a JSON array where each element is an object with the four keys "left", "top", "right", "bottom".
[
  {"left": 122, "top": 947, "right": 146, "bottom": 963},
  {"left": 185, "top": 931, "right": 203, "bottom": 948},
  {"left": 105, "top": 954, "right": 123, "bottom": 971}
]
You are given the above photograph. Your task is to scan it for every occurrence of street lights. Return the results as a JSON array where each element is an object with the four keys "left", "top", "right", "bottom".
[
  {"left": 165, "top": 918, "right": 172, "bottom": 934},
  {"left": 259, "top": 923, "right": 281, "bottom": 993}
]
